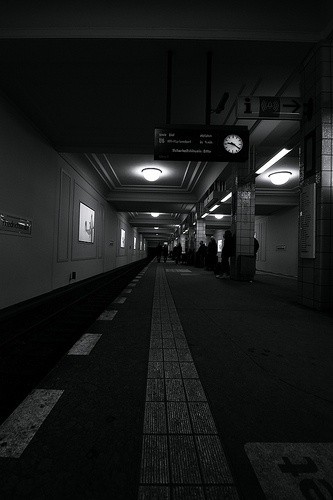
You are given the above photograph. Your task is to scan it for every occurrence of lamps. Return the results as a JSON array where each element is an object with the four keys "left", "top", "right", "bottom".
[
  {"left": 141, "top": 168, "right": 162, "bottom": 182},
  {"left": 268, "top": 172, "right": 292, "bottom": 185}
]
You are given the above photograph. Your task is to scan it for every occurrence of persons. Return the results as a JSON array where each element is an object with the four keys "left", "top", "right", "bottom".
[
  {"left": 173, "top": 242, "right": 188, "bottom": 265},
  {"left": 197, "top": 230, "right": 233, "bottom": 279},
  {"left": 151, "top": 243, "right": 168, "bottom": 263},
  {"left": 254, "top": 238, "right": 259, "bottom": 260}
]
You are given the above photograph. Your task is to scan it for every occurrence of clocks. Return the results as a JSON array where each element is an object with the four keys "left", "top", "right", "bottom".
[{"left": 223, "top": 134, "right": 243, "bottom": 154}]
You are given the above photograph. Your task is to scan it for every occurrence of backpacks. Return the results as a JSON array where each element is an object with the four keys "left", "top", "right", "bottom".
[{"left": 214, "top": 263, "right": 224, "bottom": 275}]
